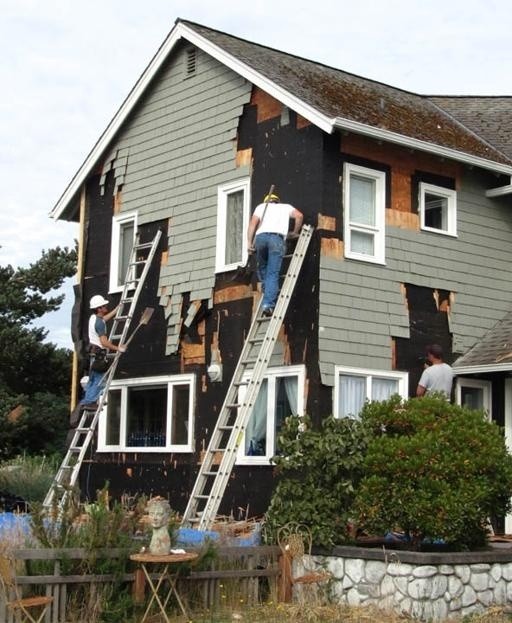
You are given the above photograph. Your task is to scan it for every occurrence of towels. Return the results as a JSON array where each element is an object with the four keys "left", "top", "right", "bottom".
[{"left": 170, "top": 548, "right": 187, "bottom": 554}]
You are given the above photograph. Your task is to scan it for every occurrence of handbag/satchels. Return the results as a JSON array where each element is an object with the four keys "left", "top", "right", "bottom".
[{"left": 91, "top": 349, "right": 109, "bottom": 374}]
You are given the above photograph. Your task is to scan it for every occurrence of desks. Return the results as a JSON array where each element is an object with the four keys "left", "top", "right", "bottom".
[{"left": 130, "top": 552, "right": 199, "bottom": 623}]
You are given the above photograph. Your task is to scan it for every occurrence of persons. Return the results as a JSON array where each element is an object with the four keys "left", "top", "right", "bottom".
[
  {"left": 248, "top": 194, "right": 304, "bottom": 317},
  {"left": 80, "top": 295, "right": 128, "bottom": 411},
  {"left": 146, "top": 497, "right": 171, "bottom": 556},
  {"left": 416, "top": 344, "right": 455, "bottom": 404}
]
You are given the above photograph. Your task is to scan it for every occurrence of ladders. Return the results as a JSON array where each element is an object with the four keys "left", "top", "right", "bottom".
[
  {"left": 38, "top": 228, "right": 165, "bottom": 537},
  {"left": 180, "top": 226, "right": 312, "bottom": 530}
]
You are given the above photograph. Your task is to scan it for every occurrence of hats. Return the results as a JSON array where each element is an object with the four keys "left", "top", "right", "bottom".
[{"left": 425, "top": 344, "right": 443, "bottom": 355}]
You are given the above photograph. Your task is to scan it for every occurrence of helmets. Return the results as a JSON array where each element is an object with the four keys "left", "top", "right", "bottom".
[
  {"left": 88, "top": 294, "right": 110, "bottom": 310},
  {"left": 262, "top": 193, "right": 280, "bottom": 203}
]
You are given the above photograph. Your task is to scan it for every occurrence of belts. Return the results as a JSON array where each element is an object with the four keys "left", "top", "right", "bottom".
[{"left": 265, "top": 232, "right": 284, "bottom": 239}]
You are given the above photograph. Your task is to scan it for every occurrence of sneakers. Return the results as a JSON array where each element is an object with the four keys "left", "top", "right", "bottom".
[
  {"left": 83, "top": 403, "right": 105, "bottom": 412},
  {"left": 262, "top": 306, "right": 273, "bottom": 317}
]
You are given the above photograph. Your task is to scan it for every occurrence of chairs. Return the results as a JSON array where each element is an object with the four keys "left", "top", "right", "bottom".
[
  {"left": 0, "top": 543, "right": 55, "bottom": 623},
  {"left": 276, "top": 520, "right": 333, "bottom": 607}
]
